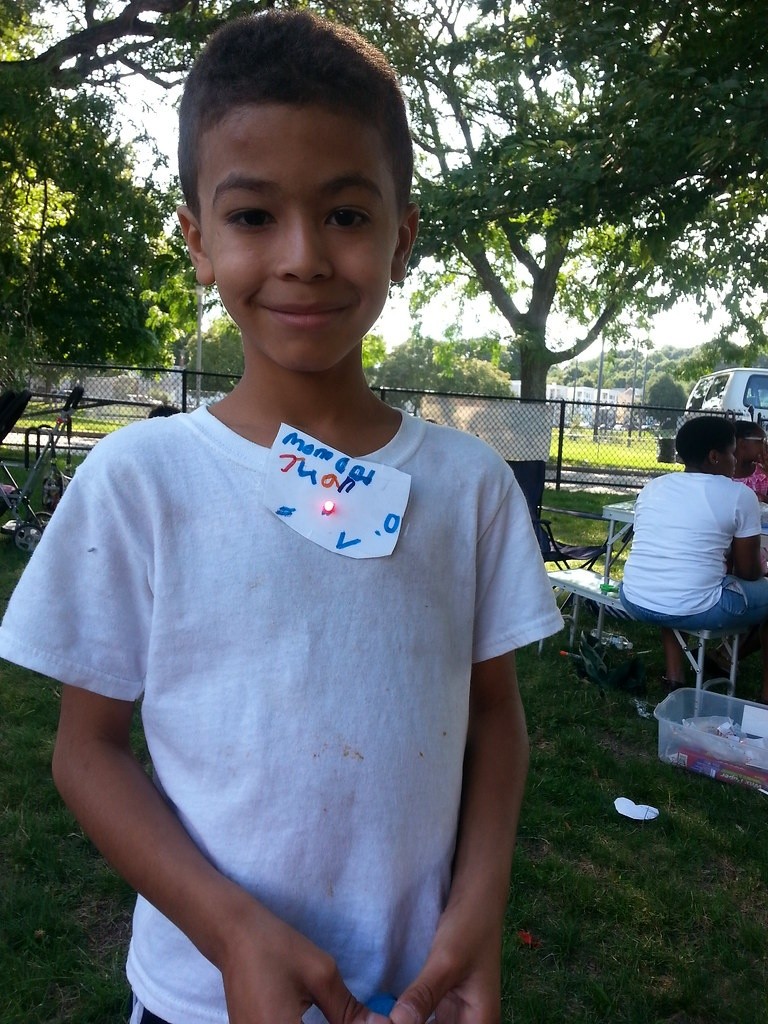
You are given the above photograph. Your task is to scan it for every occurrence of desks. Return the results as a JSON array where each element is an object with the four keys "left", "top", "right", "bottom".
[{"left": 596, "top": 500, "right": 768, "bottom": 646}]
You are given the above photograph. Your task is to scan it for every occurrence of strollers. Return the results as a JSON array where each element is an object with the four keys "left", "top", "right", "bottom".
[{"left": 0, "top": 386, "right": 86, "bottom": 551}]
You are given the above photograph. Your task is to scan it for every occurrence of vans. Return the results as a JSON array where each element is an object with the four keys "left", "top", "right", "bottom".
[{"left": 684, "top": 368, "right": 768, "bottom": 436}]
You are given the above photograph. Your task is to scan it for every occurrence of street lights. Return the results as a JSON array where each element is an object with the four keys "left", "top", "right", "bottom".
[{"left": 194, "top": 277, "right": 205, "bottom": 408}]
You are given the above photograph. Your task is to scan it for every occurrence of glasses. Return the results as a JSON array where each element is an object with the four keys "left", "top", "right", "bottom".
[{"left": 745, "top": 437, "right": 767, "bottom": 444}]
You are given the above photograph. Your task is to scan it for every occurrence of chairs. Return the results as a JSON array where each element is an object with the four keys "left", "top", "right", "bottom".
[{"left": 505, "top": 459, "right": 635, "bottom": 612}]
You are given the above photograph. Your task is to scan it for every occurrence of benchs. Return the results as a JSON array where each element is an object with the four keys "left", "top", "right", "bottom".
[{"left": 537, "top": 567, "right": 741, "bottom": 719}]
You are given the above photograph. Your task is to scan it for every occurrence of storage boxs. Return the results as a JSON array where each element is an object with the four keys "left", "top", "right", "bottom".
[{"left": 654, "top": 687, "right": 768, "bottom": 792}]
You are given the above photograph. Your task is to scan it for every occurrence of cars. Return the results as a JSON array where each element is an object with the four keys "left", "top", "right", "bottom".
[
  {"left": 127, "top": 393, "right": 163, "bottom": 406},
  {"left": 554, "top": 420, "right": 659, "bottom": 431}
]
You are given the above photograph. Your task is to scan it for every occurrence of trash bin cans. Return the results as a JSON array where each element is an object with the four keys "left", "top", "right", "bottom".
[{"left": 657, "top": 438, "right": 675, "bottom": 463}]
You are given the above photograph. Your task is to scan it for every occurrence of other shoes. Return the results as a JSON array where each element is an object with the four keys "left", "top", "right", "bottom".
[{"left": 659, "top": 666, "right": 683, "bottom": 695}]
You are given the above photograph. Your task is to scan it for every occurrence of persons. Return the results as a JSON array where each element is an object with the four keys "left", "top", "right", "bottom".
[
  {"left": 620, "top": 416, "right": 768, "bottom": 692},
  {"left": 0, "top": 6, "right": 565, "bottom": 1024}
]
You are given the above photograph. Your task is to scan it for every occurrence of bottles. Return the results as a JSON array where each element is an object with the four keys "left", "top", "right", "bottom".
[{"left": 590, "top": 629, "right": 633, "bottom": 649}]
